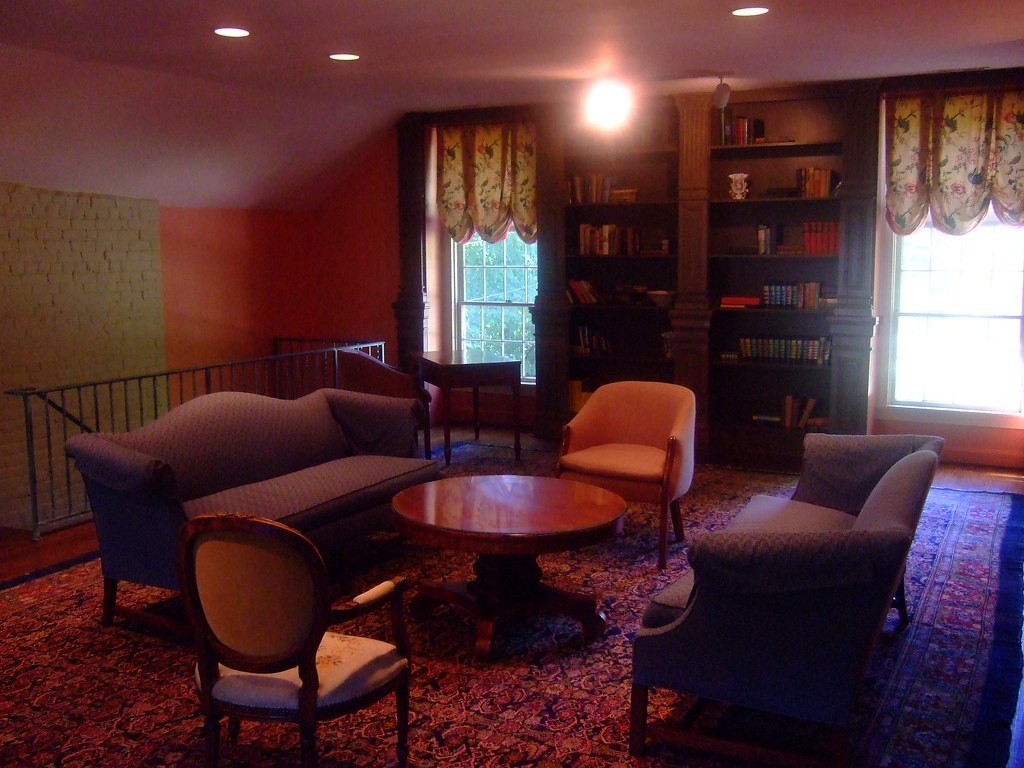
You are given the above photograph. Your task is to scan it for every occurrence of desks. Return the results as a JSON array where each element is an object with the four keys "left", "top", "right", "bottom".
[{"left": 411, "top": 349, "right": 523, "bottom": 465}]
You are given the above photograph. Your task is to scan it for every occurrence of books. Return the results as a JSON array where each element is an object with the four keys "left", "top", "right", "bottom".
[
  {"left": 758, "top": 167, "right": 847, "bottom": 197},
  {"left": 565, "top": 171, "right": 637, "bottom": 204},
  {"left": 750, "top": 392, "right": 829, "bottom": 433},
  {"left": 565, "top": 222, "right": 674, "bottom": 257},
  {"left": 569, "top": 378, "right": 594, "bottom": 412},
  {"left": 718, "top": 105, "right": 764, "bottom": 144},
  {"left": 564, "top": 275, "right": 631, "bottom": 305},
  {"left": 571, "top": 324, "right": 616, "bottom": 353},
  {"left": 720, "top": 332, "right": 832, "bottom": 365},
  {"left": 728, "top": 216, "right": 839, "bottom": 257},
  {"left": 720, "top": 282, "right": 838, "bottom": 308}
]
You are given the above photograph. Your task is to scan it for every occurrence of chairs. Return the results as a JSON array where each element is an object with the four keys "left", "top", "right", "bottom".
[
  {"left": 551, "top": 380, "right": 696, "bottom": 569},
  {"left": 339, "top": 349, "right": 432, "bottom": 460},
  {"left": 177, "top": 511, "right": 414, "bottom": 768}
]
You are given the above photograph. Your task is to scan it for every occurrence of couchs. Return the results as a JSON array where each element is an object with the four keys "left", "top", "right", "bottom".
[
  {"left": 63, "top": 387, "right": 441, "bottom": 641},
  {"left": 629, "top": 432, "right": 947, "bottom": 768}
]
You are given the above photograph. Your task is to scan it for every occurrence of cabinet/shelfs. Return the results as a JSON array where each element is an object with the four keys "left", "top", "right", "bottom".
[
  {"left": 709, "top": 141, "right": 840, "bottom": 470},
  {"left": 563, "top": 149, "right": 679, "bottom": 414}
]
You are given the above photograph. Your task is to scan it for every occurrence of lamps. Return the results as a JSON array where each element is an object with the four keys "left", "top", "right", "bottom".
[{"left": 711, "top": 76, "right": 731, "bottom": 108}]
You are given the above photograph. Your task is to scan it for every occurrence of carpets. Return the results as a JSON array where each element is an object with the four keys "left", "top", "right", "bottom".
[{"left": 0, "top": 437, "right": 1024, "bottom": 768}]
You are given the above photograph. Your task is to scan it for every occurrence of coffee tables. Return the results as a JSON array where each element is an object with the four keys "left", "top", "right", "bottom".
[{"left": 391, "top": 475, "right": 628, "bottom": 663}]
